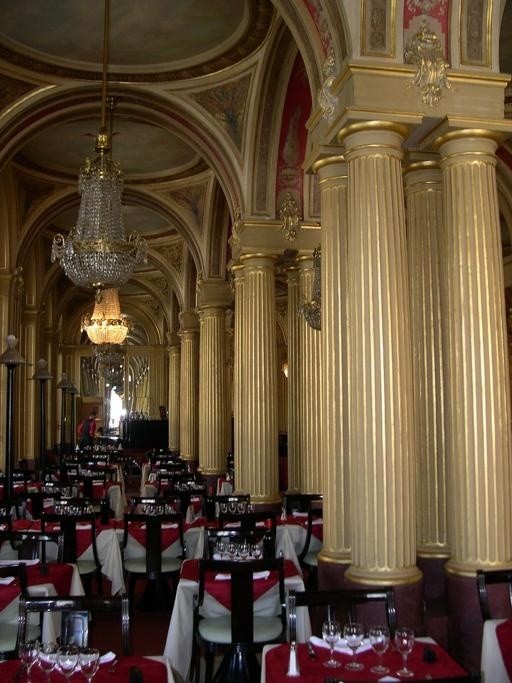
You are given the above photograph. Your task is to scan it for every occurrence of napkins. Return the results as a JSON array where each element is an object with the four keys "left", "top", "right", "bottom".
[{"left": 309, "top": 636, "right": 386, "bottom": 656}]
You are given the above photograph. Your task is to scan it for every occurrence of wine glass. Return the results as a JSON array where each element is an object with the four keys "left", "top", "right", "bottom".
[
  {"left": 1, "top": 441, "right": 206, "bottom": 532},
  {"left": 343, "top": 623, "right": 366, "bottom": 672},
  {"left": 55, "top": 644, "right": 77, "bottom": 682},
  {"left": 37, "top": 642, "right": 55, "bottom": 683},
  {"left": 367, "top": 625, "right": 392, "bottom": 675},
  {"left": 320, "top": 618, "right": 343, "bottom": 668},
  {"left": 17, "top": 639, "right": 37, "bottom": 682},
  {"left": 213, "top": 500, "right": 263, "bottom": 562},
  {"left": 77, "top": 645, "right": 101, "bottom": 683},
  {"left": 392, "top": 627, "right": 416, "bottom": 679}
]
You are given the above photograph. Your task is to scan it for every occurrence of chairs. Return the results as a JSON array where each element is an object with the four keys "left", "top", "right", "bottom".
[
  {"left": 476, "top": 568, "right": 511, "bottom": 621},
  {"left": 2, "top": 435, "right": 322, "bottom": 680},
  {"left": 288, "top": 585, "right": 398, "bottom": 639}
]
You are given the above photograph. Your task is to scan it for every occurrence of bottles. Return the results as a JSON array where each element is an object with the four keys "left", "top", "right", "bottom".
[{"left": 129, "top": 408, "right": 144, "bottom": 420}]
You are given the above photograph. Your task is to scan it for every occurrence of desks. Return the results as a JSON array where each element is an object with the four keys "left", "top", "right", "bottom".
[
  {"left": 481, "top": 619, "right": 510, "bottom": 680},
  {"left": 261, "top": 635, "right": 473, "bottom": 680}
]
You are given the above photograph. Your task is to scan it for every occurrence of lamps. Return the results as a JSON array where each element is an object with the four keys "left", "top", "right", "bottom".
[
  {"left": 51, "top": 1, "right": 148, "bottom": 303},
  {"left": 47, "top": 95, "right": 152, "bottom": 387}
]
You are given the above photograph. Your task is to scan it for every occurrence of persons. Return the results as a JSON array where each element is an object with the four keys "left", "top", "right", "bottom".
[{"left": 77, "top": 412, "right": 97, "bottom": 446}]
